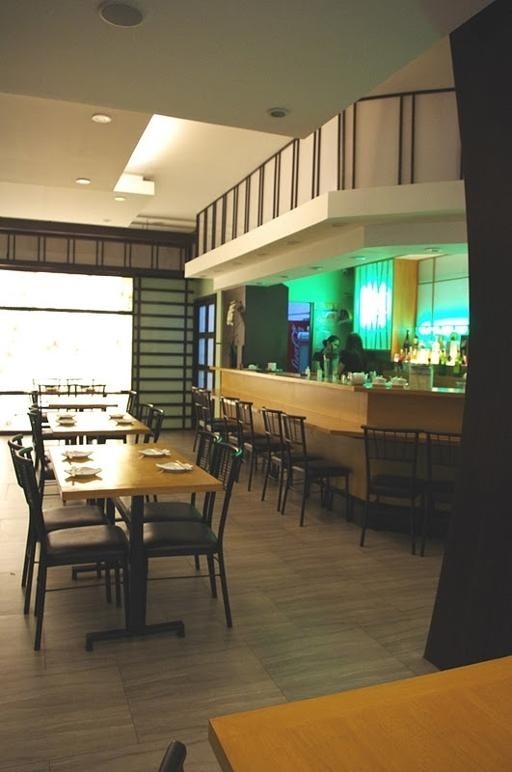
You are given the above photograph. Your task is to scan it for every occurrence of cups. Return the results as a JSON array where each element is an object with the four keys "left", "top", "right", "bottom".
[{"left": 268, "top": 362, "right": 276, "bottom": 371}]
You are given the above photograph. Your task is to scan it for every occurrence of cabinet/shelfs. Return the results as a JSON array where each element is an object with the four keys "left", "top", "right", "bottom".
[{"left": 221, "top": 370, "right": 465, "bottom": 534}]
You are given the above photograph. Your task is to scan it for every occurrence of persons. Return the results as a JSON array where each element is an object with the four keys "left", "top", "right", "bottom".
[
  {"left": 338, "top": 333, "right": 368, "bottom": 378},
  {"left": 311, "top": 335, "right": 340, "bottom": 372}
]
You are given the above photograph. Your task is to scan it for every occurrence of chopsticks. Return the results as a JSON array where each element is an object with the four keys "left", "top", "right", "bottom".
[
  {"left": 153, "top": 447, "right": 170, "bottom": 456},
  {"left": 175, "top": 459, "right": 190, "bottom": 470},
  {"left": 66, "top": 451, "right": 70, "bottom": 459},
  {"left": 72, "top": 465, "right": 76, "bottom": 478}
]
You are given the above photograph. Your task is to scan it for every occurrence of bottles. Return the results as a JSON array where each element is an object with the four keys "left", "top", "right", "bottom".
[
  {"left": 439, "top": 349, "right": 446, "bottom": 374},
  {"left": 404, "top": 336, "right": 409, "bottom": 352},
  {"left": 453, "top": 350, "right": 463, "bottom": 377}
]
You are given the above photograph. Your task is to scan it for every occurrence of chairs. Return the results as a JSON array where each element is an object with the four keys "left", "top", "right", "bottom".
[
  {"left": 276, "top": 414, "right": 352, "bottom": 527},
  {"left": 420, "top": 430, "right": 463, "bottom": 557},
  {"left": 73, "top": 384, "right": 107, "bottom": 412},
  {"left": 191, "top": 387, "right": 226, "bottom": 457},
  {"left": 38, "top": 384, "right": 72, "bottom": 411},
  {"left": 86, "top": 390, "right": 138, "bottom": 444},
  {"left": 7, "top": 434, "right": 113, "bottom": 615},
  {"left": 262, "top": 408, "right": 310, "bottom": 512},
  {"left": 196, "top": 389, "right": 238, "bottom": 469},
  {"left": 13, "top": 446, "right": 131, "bottom": 650},
  {"left": 143, "top": 441, "right": 244, "bottom": 629},
  {"left": 142, "top": 431, "right": 224, "bottom": 570},
  {"left": 360, "top": 425, "right": 422, "bottom": 554},
  {"left": 220, "top": 397, "right": 245, "bottom": 478},
  {"left": 27, "top": 411, "right": 95, "bottom": 507},
  {"left": 235, "top": 401, "right": 285, "bottom": 492},
  {"left": 143, "top": 406, "right": 164, "bottom": 503},
  {"left": 135, "top": 403, "right": 154, "bottom": 444},
  {"left": 28, "top": 404, "right": 69, "bottom": 471},
  {"left": 31, "top": 390, "right": 48, "bottom": 423}
]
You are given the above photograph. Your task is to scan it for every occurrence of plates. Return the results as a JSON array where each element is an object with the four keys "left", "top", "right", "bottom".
[
  {"left": 55, "top": 419, "right": 78, "bottom": 424},
  {"left": 138, "top": 448, "right": 171, "bottom": 457},
  {"left": 64, "top": 466, "right": 102, "bottom": 477},
  {"left": 109, "top": 414, "right": 125, "bottom": 417},
  {"left": 61, "top": 450, "right": 95, "bottom": 457},
  {"left": 156, "top": 462, "right": 193, "bottom": 472},
  {"left": 114, "top": 419, "right": 137, "bottom": 423},
  {"left": 56, "top": 414, "right": 77, "bottom": 417}
]
